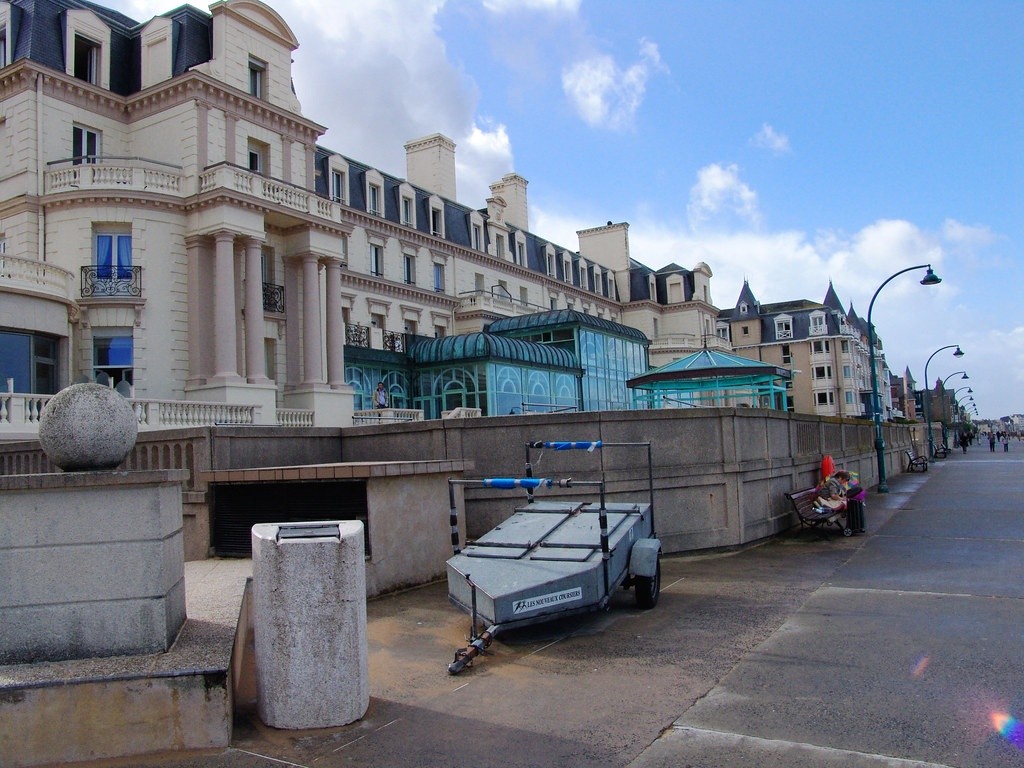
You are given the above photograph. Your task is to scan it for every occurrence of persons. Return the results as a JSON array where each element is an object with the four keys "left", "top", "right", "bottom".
[
  {"left": 959, "top": 432, "right": 969, "bottom": 454},
  {"left": 373, "top": 381, "right": 388, "bottom": 420},
  {"left": 968, "top": 431, "right": 981, "bottom": 446},
  {"left": 812, "top": 470, "right": 866, "bottom": 535},
  {"left": 1002, "top": 431, "right": 1009, "bottom": 452},
  {"left": 987, "top": 432, "right": 996, "bottom": 452},
  {"left": 996, "top": 431, "right": 1001, "bottom": 442}
]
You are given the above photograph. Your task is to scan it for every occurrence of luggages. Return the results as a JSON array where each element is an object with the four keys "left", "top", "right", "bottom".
[{"left": 844, "top": 497, "right": 866, "bottom": 536}]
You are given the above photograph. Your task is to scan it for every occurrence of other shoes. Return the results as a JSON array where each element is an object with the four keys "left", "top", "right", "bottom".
[{"left": 963, "top": 450, "right": 967, "bottom": 454}]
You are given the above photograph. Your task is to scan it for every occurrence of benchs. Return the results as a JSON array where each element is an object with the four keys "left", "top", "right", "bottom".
[
  {"left": 932, "top": 444, "right": 947, "bottom": 459},
  {"left": 906, "top": 449, "right": 928, "bottom": 471},
  {"left": 941, "top": 444, "right": 952, "bottom": 454},
  {"left": 784, "top": 485, "right": 847, "bottom": 530}
]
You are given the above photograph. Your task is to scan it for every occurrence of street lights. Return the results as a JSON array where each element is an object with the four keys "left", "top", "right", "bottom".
[
  {"left": 925, "top": 345, "right": 965, "bottom": 462},
  {"left": 868, "top": 263, "right": 942, "bottom": 493},
  {"left": 943, "top": 371, "right": 970, "bottom": 451},
  {"left": 949, "top": 387, "right": 973, "bottom": 448},
  {"left": 956, "top": 395, "right": 978, "bottom": 447}
]
